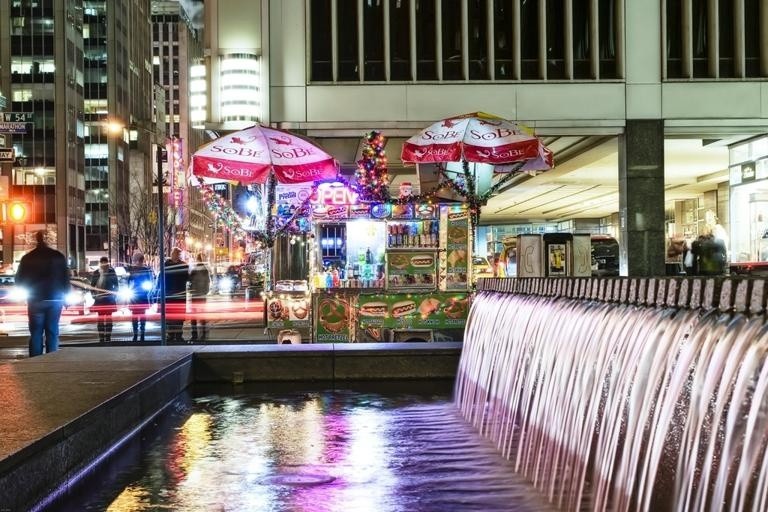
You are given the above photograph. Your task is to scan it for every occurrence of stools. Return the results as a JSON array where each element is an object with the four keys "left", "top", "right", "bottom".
[{"left": 277, "top": 330, "right": 302, "bottom": 344}]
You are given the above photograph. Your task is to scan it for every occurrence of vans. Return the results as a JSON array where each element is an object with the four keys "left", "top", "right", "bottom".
[
  {"left": 590, "top": 235, "right": 619, "bottom": 273},
  {"left": 497, "top": 235, "right": 519, "bottom": 278}
]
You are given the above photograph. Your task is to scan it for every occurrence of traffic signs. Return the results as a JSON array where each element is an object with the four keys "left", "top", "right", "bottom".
[{"left": 0, "top": 92, "right": 36, "bottom": 165}]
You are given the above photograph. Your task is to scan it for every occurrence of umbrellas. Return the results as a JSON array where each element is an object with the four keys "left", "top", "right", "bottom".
[
  {"left": 186, "top": 120, "right": 340, "bottom": 329},
  {"left": 401, "top": 111, "right": 555, "bottom": 289}
]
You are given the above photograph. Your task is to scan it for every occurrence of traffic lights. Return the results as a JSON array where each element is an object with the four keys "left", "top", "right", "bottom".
[{"left": 9, "top": 201, "right": 34, "bottom": 224}]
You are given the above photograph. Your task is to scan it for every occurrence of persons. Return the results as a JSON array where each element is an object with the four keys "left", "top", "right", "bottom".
[
  {"left": 398, "top": 181, "right": 414, "bottom": 198},
  {"left": 154, "top": 246, "right": 189, "bottom": 343},
  {"left": 14, "top": 229, "right": 71, "bottom": 357},
  {"left": 88, "top": 255, "right": 118, "bottom": 344},
  {"left": 123, "top": 252, "right": 154, "bottom": 342},
  {"left": 188, "top": 252, "right": 210, "bottom": 339},
  {"left": 663, "top": 229, "right": 730, "bottom": 278}
]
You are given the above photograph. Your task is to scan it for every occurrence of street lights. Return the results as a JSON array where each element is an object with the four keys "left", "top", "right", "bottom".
[{"left": 100, "top": 116, "right": 166, "bottom": 342}]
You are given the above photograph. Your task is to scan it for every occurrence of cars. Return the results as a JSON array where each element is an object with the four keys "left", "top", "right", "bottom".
[
  {"left": 472, "top": 256, "right": 494, "bottom": 284},
  {"left": 0, "top": 258, "right": 241, "bottom": 305}
]
